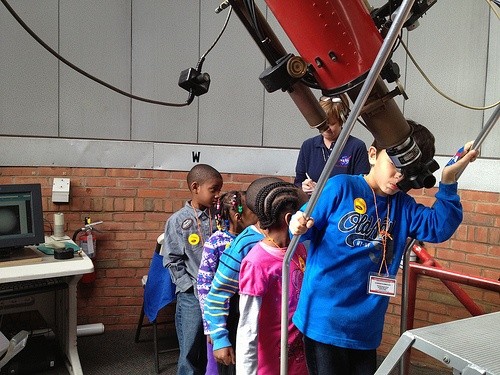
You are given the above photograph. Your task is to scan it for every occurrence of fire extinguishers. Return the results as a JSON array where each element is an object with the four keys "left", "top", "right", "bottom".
[{"left": 73, "top": 217, "right": 103, "bottom": 285}]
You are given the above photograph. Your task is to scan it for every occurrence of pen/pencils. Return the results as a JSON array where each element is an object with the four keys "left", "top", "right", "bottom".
[{"left": 306, "top": 172, "right": 315, "bottom": 188}]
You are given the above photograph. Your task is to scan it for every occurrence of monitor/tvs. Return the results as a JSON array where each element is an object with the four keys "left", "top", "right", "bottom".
[{"left": 0, "top": 183, "right": 45, "bottom": 262}]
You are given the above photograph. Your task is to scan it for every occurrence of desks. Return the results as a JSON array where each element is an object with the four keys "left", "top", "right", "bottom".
[{"left": 0, "top": 236, "right": 95, "bottom": 375}]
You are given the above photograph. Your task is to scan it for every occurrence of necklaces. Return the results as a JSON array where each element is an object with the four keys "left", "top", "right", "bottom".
[
  {"left": 191, "top": 201, "right": 212, "bottom": 247},
  {"left": 267, "top": 234, "right": 304, "bottom": 274}
]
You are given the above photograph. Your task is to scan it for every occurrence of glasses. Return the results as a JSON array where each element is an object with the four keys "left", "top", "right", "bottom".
[{"left": 319, "top": 96, "right": 345, "bottom": 112}]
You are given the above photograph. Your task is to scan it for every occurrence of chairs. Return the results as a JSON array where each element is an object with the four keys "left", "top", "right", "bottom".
[{"left": 134, "top": 232, "right": 180, "bottom": 375}]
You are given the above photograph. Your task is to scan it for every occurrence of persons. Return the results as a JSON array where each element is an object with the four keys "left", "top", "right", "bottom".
[
  {"left": 236, "top": 182, "right": 309, "bottom": 375},
  {"left": 289, "top": 118, "right": 479, "bottom": 375},
  {"left": 196, "top": 190, "right": 257, "bottom": 375},
  {"left": 203, "top": 176, "right": 286, "bottom": 375},
  {"left": 294, "top": 94, "right": 372, "bottom": 197},
  {"left": 161, "top": 164, "right": 223, "bottom": 375}
]
